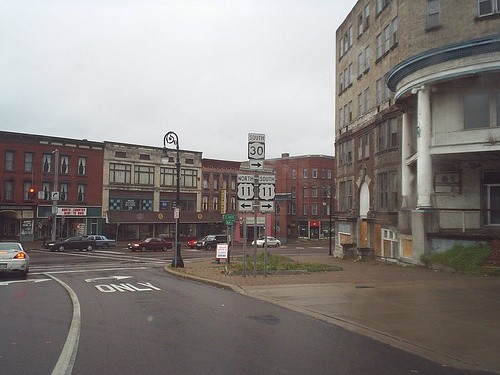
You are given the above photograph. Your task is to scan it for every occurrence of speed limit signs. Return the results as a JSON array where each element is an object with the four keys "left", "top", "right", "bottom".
[{"left": 248, "top": 142, "right": 265, "bottom": 160}]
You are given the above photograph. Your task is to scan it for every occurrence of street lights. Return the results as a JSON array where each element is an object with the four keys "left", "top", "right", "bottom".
[
  {"left": 322, "top": 188, "right": 333, "bottom": 255},
  {"left": 160, "top": 130, "right": 184, "bottom": 269}
]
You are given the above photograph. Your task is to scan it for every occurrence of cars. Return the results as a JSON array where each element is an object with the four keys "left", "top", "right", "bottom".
[
  {"left": 0, "top": 241, "right": 31, "bottom": 277},
  {"left": 41, "top": 234, "right": 116, "bottom": 252},
  {"left": 251, "top": 236, "right": 282, "bottom": 248},
  {"left": 127, "top": 237, "right": 174, "bottom": 252}
]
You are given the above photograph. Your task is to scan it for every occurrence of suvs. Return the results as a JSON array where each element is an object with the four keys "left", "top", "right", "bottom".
[
  {"left": 187, "top": 233, "right": 213, "bottom": 249},
  {"left": 196, "top": 234, "right": 231, "bottom": 251}
]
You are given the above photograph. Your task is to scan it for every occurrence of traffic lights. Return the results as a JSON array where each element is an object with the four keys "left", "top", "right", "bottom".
[
  {"left": 28, "top": 188, "right": 37, "bottom": 199},
  {"left": 277, "top": 207, "right": 280, "bottom": 213},
  {"left": 48, "top": 216, "right": 52, "bottom": 222}
]
